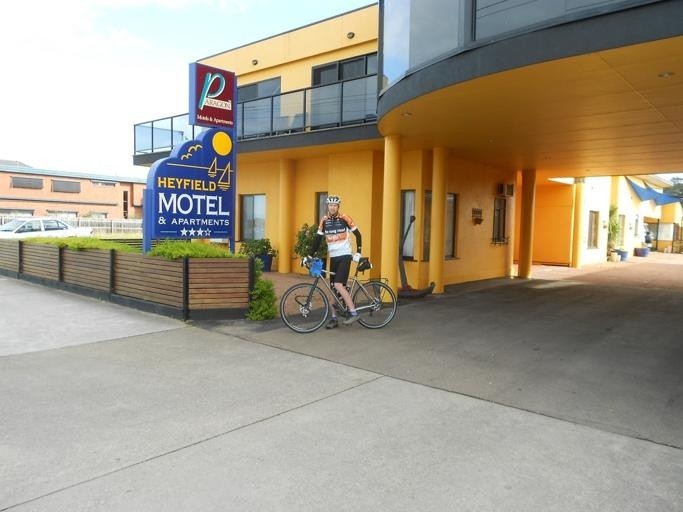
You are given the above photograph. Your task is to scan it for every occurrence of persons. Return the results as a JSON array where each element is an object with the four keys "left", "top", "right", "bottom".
[{"left": 299, "top": 194, "right": 361, "bottom": 329}]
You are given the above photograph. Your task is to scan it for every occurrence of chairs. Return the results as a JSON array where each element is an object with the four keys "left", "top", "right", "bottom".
[{"left": 273, "top": 111, "right": 308, "bottom": 136}]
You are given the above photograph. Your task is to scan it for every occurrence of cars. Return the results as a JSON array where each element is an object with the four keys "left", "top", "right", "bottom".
[{"left": 0, "top": 215, "right": 92, "bottom": 239}]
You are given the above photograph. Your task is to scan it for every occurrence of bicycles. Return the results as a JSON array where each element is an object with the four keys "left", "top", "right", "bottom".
[{"left": 278, "top": 252, "right": 398, "bottom": 334}]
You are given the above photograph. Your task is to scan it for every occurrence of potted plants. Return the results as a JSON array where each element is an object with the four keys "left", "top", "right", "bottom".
[
  {"left": 239, "top": 238, "right": 278, "bottom": 272},
  {"left": 294, "top": 223, "right": 327, "bottom": 278}
]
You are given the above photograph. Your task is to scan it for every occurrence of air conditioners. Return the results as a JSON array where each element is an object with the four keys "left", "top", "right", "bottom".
[{"left": 497, "top": 182, "right": 514, "bottom": 197}]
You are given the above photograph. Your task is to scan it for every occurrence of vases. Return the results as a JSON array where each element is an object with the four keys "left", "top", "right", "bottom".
[
  {"left": 635, "top": 248, "right": 648, "bottom": 257},
  {"left": 617, "top": 251, "right": 629, "bottom": 261}
]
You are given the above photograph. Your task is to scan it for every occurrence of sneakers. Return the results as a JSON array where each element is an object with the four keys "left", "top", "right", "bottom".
[
  {"left": 326, "top": 319, "right": 338, "bottom": 329},
  {"left": 342, "top": 313, "right": 360, "bottom": 325}
]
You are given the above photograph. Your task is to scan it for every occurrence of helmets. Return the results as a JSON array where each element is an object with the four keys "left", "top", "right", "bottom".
[{"left": 326, "top": 196, "right": 340, "bottom": 204}]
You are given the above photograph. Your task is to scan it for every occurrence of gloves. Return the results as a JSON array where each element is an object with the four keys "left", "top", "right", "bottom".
[
  {"left": 300, "top": 255, "right": 313, "bottom": 266},
  {"left": 353, "top": 253, "right": 361, "bottom": 262}
]
